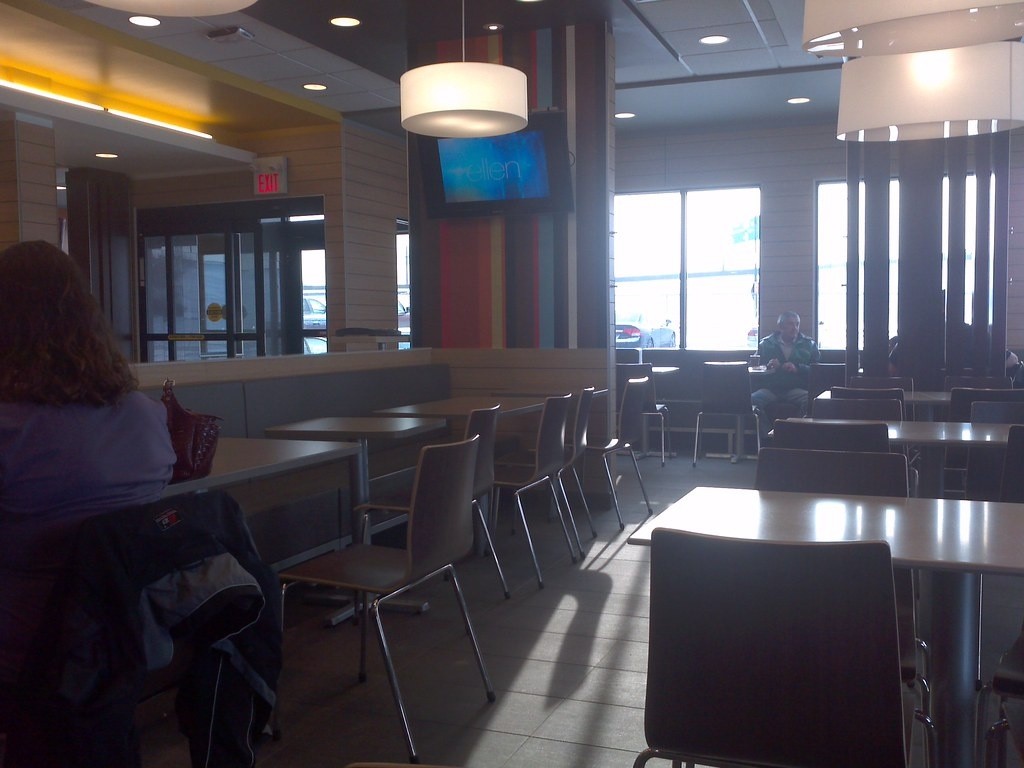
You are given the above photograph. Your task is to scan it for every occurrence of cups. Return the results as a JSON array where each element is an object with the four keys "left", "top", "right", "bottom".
[{"left": 750, "top": 355, "right": 760, "bottom": 369}]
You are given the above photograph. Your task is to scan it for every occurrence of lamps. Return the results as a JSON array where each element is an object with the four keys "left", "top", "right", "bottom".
[
  {"left": 803, "top": 0, "right": 1024, "bottom": 142},
  {"left": 84, "top": 0, "right": 260, "bottom": 17},
  {"left": 401, "top": 0, "right": 528, "bottom": 138}
]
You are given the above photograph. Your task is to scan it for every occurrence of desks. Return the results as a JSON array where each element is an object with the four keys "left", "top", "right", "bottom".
[
  {"left": 265, "top": 416, "right": 446, "bottom": 626},
  {"left": 813, "top": 390, "right": 951, "bottom": 422},
  {"left": 768, "top": 417, "right": 1024, "bottom": 502},
  {"left": 155, "top": 436, "right": 359, "bottom": 491},
  {"left": 628, "top": 486, "right": 1024, "bottom": 768},
  {"left": 373, "top": 395, "right": 544, "bottom": 421},
  {"left": 652, "top": 367, "right": 680, "bottom": 375}
]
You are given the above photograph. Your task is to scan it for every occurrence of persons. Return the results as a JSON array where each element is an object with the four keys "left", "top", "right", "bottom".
[
  {"left": 889, "top": 322, "right": 1018, "bottom": 377},
  {"left": 0, "top": 240, "right": 177, "bottom": 692},
  {"left": 751, "top": 311, "right": 821, "bottom": 435}
]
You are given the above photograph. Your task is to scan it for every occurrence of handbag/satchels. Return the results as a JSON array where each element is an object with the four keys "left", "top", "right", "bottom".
[{"left": 161, "top": 377, "right": 225, "bottom": 483}]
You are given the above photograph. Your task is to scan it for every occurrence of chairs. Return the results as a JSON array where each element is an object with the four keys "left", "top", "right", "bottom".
[{"left": 275, "top": 361, "right": 1024, "bottom": 768}]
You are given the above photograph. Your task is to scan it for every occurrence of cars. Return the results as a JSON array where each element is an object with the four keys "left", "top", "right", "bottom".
[
  {"left": 747, "top": 318, "right": 824, "bottom": 351},
  {"left": 614, "top": 312, "right": 676, "bottom": 349},
  {"left": 301, "top": 297, "right": 327, "bottom": 330},
  {"left": 398, "top": 300, "right": 411, "bottom": 329}
]
